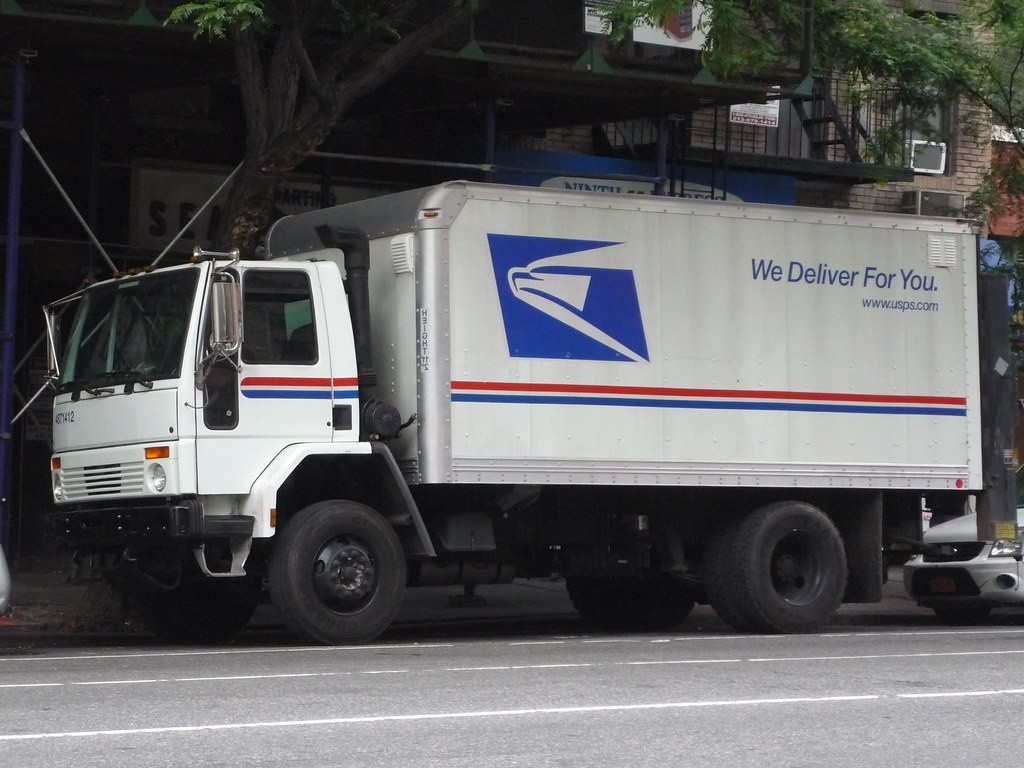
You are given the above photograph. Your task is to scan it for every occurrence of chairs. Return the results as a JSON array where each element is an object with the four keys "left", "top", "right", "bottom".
[{"left": 282, "top": 324, "right": 314, "bottom": 361}]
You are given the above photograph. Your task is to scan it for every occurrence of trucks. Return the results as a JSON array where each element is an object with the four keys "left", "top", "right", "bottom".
[{"left": 42, "top": 178, "right": 985, "bottom": 647}]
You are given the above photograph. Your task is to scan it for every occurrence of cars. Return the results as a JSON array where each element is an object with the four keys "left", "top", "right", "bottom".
[{"left": 903, "top": 464, "right": 1024, "bottom": 626}]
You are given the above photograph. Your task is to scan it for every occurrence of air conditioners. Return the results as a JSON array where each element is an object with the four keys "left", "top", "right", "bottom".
[
  {"left": 903, "top": 189, "right": 966, "bottom": 221},
  {"left": 905, "top": 141, "right": 947, "bottom": 174}
]
